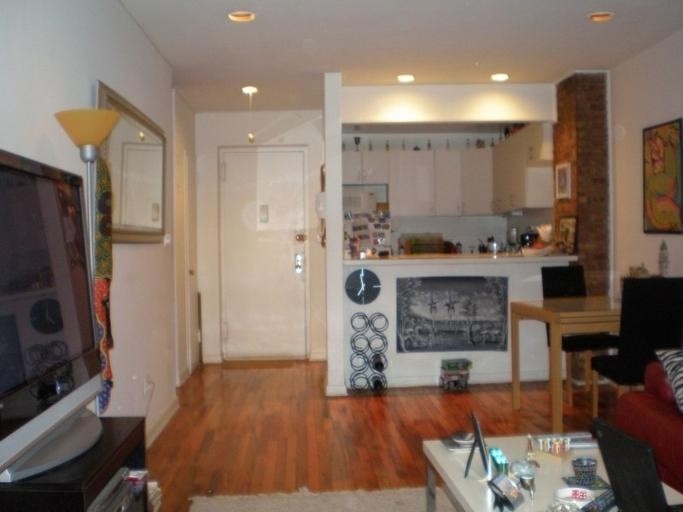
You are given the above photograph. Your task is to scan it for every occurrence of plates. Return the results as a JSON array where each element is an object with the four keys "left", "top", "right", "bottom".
[{"left": 440, "top": 436, "right": 487, "bottom": 456}]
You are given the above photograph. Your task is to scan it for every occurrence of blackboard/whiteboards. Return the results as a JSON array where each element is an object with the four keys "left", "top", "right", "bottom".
[{"left": 396, "top": 278, "right": 508, "bottom": 351}]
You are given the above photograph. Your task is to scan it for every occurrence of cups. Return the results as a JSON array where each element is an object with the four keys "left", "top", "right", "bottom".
[{"left": 571, "top": 457, "right": 598, "bottom": 486}]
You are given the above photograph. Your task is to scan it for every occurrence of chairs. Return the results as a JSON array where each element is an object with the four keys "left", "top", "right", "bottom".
[
  {"left": 590, "top": 276, "right": 683, "bottom": 422},
  {"left": 541, "top": 265, "right": 620, "bottom": 407}
]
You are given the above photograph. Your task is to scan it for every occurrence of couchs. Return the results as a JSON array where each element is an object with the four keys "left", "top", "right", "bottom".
[{"left": 614, "top": 361, "right": 683, "bottom": 493}]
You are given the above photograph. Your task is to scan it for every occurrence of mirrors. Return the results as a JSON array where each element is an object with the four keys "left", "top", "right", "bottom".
[{"left": 93, "top": 79, "right": 169, "bottom": 242}]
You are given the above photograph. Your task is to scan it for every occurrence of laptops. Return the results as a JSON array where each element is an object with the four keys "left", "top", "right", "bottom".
[{"left": 592, "top": 418, "right": 683, "bottom": 511}]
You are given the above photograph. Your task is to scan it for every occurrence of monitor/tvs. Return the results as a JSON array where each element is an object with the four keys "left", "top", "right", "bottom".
[{"left": 0, "top": 147, "right": 104, "bottom": 484}]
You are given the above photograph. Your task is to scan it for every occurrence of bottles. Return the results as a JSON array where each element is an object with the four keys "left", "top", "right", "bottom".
[{"left": 658, "top": 239, "right": 670, "bottom": 277}]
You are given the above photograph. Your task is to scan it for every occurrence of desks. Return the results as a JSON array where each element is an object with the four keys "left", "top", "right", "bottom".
[{"left": 510, "top": 295, "right": 620, "bottom": 434}]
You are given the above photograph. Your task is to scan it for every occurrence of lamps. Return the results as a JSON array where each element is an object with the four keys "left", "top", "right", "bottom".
[{"left": 54, "top": 109, "right": 122, "bottom": 419}]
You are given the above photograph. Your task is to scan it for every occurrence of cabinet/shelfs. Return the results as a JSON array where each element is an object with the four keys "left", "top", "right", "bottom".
[
  {"left": 0, "top": 416, "right": 149, "bottom": 512},
  {"left": 342, "top": 122, "right": 555, "bottom": 217}
]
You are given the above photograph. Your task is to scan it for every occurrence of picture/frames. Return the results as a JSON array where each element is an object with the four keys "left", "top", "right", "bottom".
[
  {"left": 642, "top": 118, "right": 683, "bottom": 235},
  {"left": 558, "top": 216, "right": 578, "bottom": 254},
  {"left": 554, "top": 162, "right": 572, "bottom": 200}
]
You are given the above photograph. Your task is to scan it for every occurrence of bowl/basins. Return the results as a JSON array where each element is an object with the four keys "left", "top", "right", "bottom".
[{"left": 449, "top": 431, "right": 476, "bottom": 448}]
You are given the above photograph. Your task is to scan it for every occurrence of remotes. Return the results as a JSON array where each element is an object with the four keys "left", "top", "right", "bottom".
[{"left": 581, "top": 489, "right": 615, "bottom": 512}]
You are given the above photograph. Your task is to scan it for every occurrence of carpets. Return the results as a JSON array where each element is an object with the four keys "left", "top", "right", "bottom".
[{"left": 188, "top": 485, "right": 457, "bottom": 512}]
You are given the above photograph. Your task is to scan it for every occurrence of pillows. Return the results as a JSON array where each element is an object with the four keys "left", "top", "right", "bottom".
[{"left": 653, "top": 349, "right": 683, "bottom": 415}]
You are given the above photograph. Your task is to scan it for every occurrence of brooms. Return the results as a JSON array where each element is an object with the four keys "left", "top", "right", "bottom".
[{"left": 55, "top": 108, "right": 121, "bottom": 282}]
